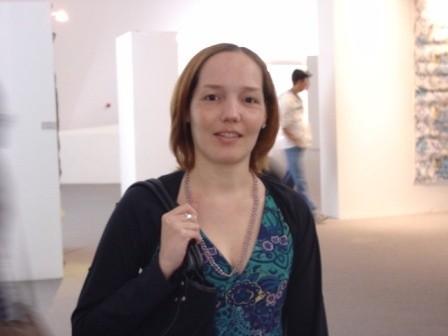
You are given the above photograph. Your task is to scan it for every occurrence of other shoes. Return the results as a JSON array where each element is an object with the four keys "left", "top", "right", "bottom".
[{"left": 313, "top": 214, "right": 328, "bottom": 224}]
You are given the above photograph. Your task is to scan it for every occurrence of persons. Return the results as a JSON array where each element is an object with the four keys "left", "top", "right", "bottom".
[
  {"left": 276, "top": 68, "right": 328, "bottom": 224},
  {"left": 68, "top": 42, "right": 331, "bottom": 336}
]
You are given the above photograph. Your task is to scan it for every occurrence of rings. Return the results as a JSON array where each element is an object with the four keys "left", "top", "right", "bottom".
[{"left": 184, "top": 213, "right": 192, "bottom": 222}]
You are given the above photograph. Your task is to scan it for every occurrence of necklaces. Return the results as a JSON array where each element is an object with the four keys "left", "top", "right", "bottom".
[{"left": 183, "top": 165, "right": 259, "bottom": 276}]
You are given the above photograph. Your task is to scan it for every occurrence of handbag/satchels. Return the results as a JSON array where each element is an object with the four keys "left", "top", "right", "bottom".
[{"left": 113, "top": 179, "right": 217, "bottom": 336}]
